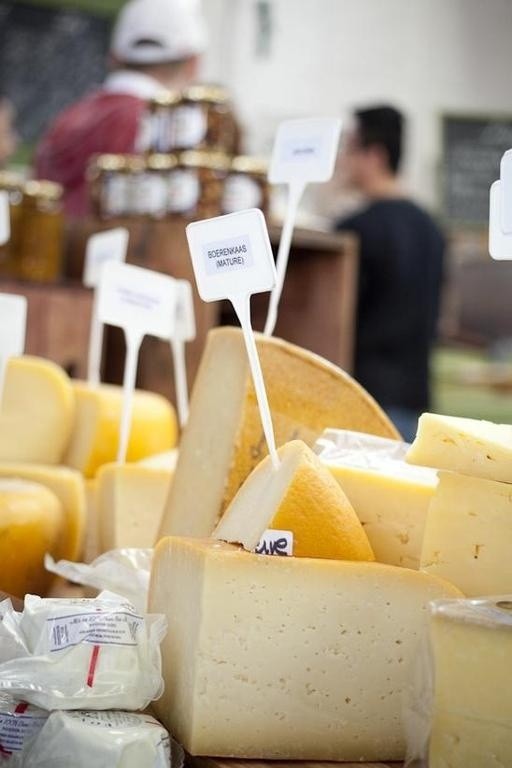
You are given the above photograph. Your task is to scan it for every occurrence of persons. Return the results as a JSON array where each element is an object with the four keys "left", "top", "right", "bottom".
[
  {"left": 325, "top": 103, "right": 448, "bottom": 441},
  {"left": 31, "top": 0, "right": 272, "bottom": 212}
]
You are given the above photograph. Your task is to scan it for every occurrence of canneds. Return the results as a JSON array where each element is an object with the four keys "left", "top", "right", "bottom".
[
  {"left": 0, "top": 169, "right": 65, "bottom": 287},
  {"left": 87, "top": 85, "right": 269, "bottom": 222}
]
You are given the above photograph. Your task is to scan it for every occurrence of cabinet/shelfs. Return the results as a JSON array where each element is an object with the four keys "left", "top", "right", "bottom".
[{"left": 0, "top": 214, "right": 359, "bottom": 440}]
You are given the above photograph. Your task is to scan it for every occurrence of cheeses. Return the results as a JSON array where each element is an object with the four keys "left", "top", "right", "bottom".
[{"left": 0, "top": 327, "right": 512, "bottom": 768}]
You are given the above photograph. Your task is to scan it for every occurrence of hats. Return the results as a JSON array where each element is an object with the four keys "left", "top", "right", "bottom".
[
  {"left": 108, "top": 0, "right": 204, "bottom": 65},
  {"left": 352, "top": 173, "right": 356, "bottom": 176}
]
